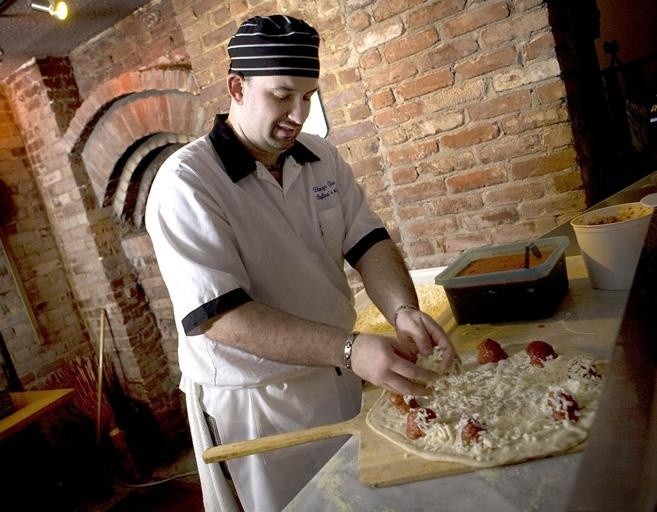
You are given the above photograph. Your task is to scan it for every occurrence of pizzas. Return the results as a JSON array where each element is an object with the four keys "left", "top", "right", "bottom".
[{"left": 365, "top": 338, "right": 606, "bottom": 469}]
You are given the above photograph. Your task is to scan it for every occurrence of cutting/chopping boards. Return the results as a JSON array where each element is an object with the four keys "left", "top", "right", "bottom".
[{"left": 200, "top": 341, "right": 592, "bottom": 491}]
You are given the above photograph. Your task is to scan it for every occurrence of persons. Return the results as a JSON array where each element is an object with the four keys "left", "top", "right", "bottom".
[{"left": 144, "top": 14, "right": 461, "bottom": 512}]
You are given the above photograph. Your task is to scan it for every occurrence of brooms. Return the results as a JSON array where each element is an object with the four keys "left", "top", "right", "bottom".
[{"left": 34, "top": 308, "right": 135, "bottom": 482}]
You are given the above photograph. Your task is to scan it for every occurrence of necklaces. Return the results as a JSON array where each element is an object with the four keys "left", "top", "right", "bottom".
[{"left": 266, "top": 162, "right": 279, "bottom": 172}]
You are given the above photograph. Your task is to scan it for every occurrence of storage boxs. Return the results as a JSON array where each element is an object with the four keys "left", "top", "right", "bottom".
[{"left": 434, "top": 236, "right": 570, "bottom": 324}]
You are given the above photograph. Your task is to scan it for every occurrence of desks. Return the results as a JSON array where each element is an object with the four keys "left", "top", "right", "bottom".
[{"left": 0, "top": 383, "right": 76, "bottom": 438}]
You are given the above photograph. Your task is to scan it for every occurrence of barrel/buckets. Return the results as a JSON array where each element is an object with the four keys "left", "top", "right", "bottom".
[{"left": 571, "top": 202, "right": 656, "bottom": 290}]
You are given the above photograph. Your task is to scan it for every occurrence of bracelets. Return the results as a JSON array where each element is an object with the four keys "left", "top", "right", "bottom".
[
  {"left": 343, "top": 331, "right": 359, "bottom": 369},
  {"left": 393, "top": 305, "right": 419, "bottom": 327}
]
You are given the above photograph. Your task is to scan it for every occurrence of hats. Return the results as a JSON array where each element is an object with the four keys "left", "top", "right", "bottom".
[{"left": 228, "top": 13, "right": 320, "bottom": 79}]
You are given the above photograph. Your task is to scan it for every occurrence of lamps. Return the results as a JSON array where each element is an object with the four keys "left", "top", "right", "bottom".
[{"left": 29, "top": 0, "right": 69, "bottom": 20}]
